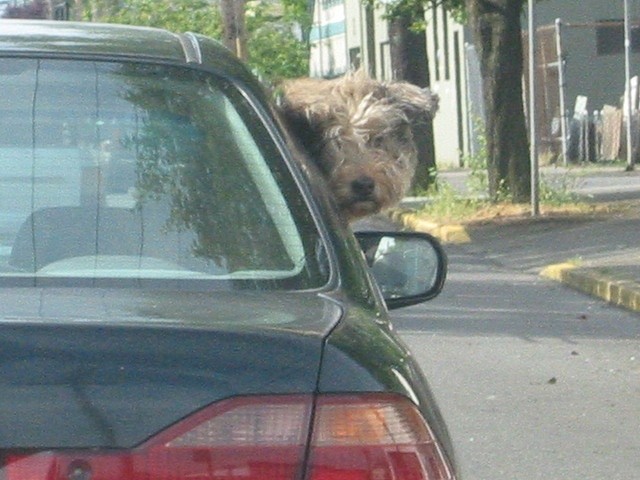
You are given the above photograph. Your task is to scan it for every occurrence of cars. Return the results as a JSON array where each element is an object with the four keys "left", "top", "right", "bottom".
[{"left": 0, "top": 17, "right": 459, "bottom": 480}]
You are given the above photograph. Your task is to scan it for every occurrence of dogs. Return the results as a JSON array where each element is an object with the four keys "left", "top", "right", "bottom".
[{"left": 269, "top": 62, "right": 441, "bottom": 223}]
